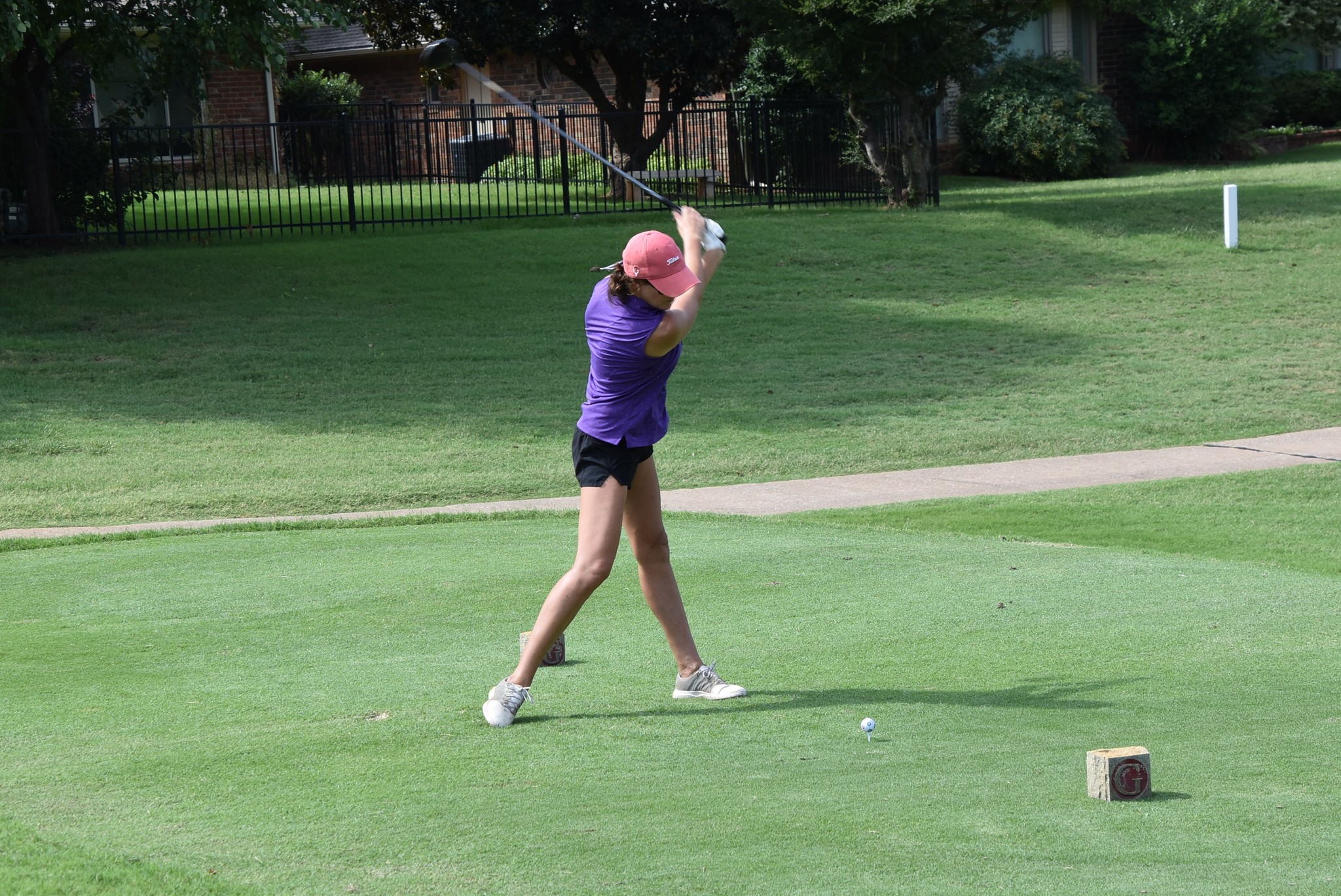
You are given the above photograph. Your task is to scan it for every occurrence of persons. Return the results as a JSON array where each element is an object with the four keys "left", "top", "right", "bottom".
[{"left": 483, "top": 206, "right": 748, "bottom": 729}]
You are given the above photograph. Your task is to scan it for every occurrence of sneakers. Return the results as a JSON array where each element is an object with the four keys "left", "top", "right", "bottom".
[
  {"left": 482, "top": 677, "right": 534, "bottom": 728},
  {"left": 672, "top": 658, "right": 747, "bottom": 700}
]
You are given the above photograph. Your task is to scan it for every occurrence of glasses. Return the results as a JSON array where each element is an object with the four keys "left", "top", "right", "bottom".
[{"left": 644, "top": 279, "right": 663, "bottom": 295}]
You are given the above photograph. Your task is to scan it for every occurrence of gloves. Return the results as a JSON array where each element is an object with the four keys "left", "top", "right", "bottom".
[{"left": 700, "top": 217, "right": 727, "bottom": 254}]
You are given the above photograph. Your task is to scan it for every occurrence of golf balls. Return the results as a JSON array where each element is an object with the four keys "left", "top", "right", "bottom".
[{"left": 861, "top": 718, "right": 876, "bottom": 732}]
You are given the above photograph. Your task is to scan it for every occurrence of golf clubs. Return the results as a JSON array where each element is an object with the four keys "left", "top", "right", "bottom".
[{"left": 417, "top": 37, "right": 729, "bottom": 246}]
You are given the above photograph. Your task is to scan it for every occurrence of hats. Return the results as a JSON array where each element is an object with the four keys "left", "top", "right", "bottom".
[{"left": 622, "top": 230, "right": 702, "bottom": 297}]
